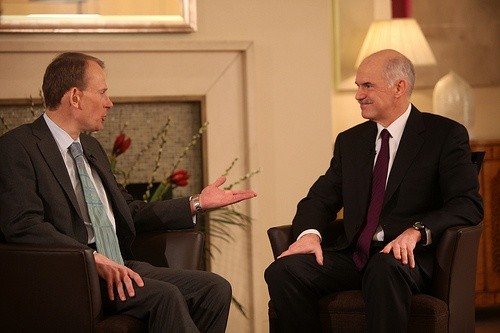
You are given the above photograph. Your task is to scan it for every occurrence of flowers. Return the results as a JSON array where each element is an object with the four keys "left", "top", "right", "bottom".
[{"left": 109, "top": 129, "right": 192, "bottom": 203}]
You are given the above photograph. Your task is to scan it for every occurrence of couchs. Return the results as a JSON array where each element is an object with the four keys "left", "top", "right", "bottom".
[
  {"left": 0, "top": 229, "right": 205, "bottom": 333},
  {"left": 267, "top": 151, "right": 485, "bottom": 333}
]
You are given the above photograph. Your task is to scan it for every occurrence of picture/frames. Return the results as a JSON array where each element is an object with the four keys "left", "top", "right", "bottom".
[{"left": 0, "top": 0, "right": 198, "bottom": 33}]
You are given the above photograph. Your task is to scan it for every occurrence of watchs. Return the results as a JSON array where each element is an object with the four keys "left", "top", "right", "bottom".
[{"left": 412, "top": 221, "right": 427, "bottom": 244}]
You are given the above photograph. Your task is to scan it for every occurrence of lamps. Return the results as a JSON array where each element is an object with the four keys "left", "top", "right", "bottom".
[{"left": 353, "top": 18, "right": 436, "bottom": 70}]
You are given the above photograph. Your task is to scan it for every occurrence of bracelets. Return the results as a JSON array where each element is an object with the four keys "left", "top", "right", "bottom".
[{"left": 193, "top": 194, "right": 207, "bottom": 214}]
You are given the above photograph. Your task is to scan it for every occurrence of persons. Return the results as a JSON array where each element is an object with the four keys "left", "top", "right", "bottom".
[
  {"left": 0, "top": 51, "right": 258, "bottom": 333},
  {"left": 264, "top": 49, "right": 485, "bottom": 333}
]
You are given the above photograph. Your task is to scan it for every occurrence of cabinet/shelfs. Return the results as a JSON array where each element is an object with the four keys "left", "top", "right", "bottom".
[{"left": 468, "top": 138, "right": 500, "bottom": 307}]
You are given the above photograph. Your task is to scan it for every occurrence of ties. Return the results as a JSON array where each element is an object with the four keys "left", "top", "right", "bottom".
[
  {"left": 68, "top": 142, "right": 125, "bottom": 266},
  {"left": 354, "top": 128, "right": 393, "bottom": 270}
]
